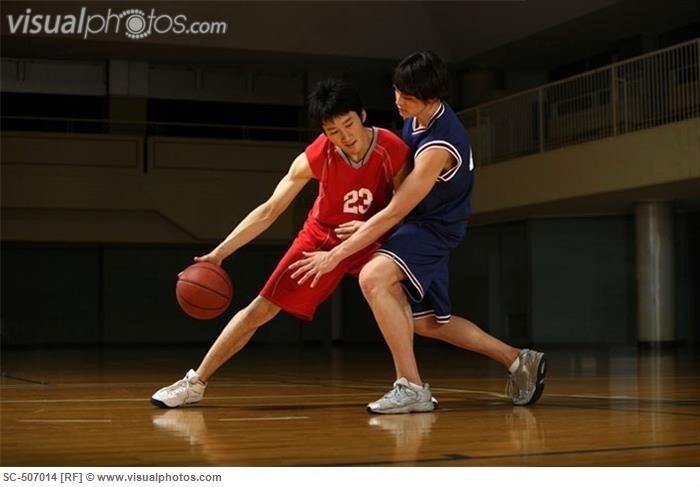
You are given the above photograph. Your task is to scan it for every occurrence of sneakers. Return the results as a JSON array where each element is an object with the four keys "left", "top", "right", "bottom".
[
  {"left": 367, "top": 377, "right": 434, "bottom": 413},
  {"left": 151, "top": 369, "right": 208, "bottom": 408},
  {"left": 508, "top": 348, "right": 546, "bottom": 405}
]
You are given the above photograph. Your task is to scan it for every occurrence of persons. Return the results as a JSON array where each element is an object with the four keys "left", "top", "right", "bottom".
[
  {"left": 288, "top": 48, "right": 547, "bottom": 416},
  {"left": 148, "top": 79, "right": 414, "bottom": 408}
]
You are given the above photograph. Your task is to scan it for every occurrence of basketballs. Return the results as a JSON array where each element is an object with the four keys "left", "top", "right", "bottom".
[{"left": 176, "top": 262, "right": 233, "bottom": 320}]
